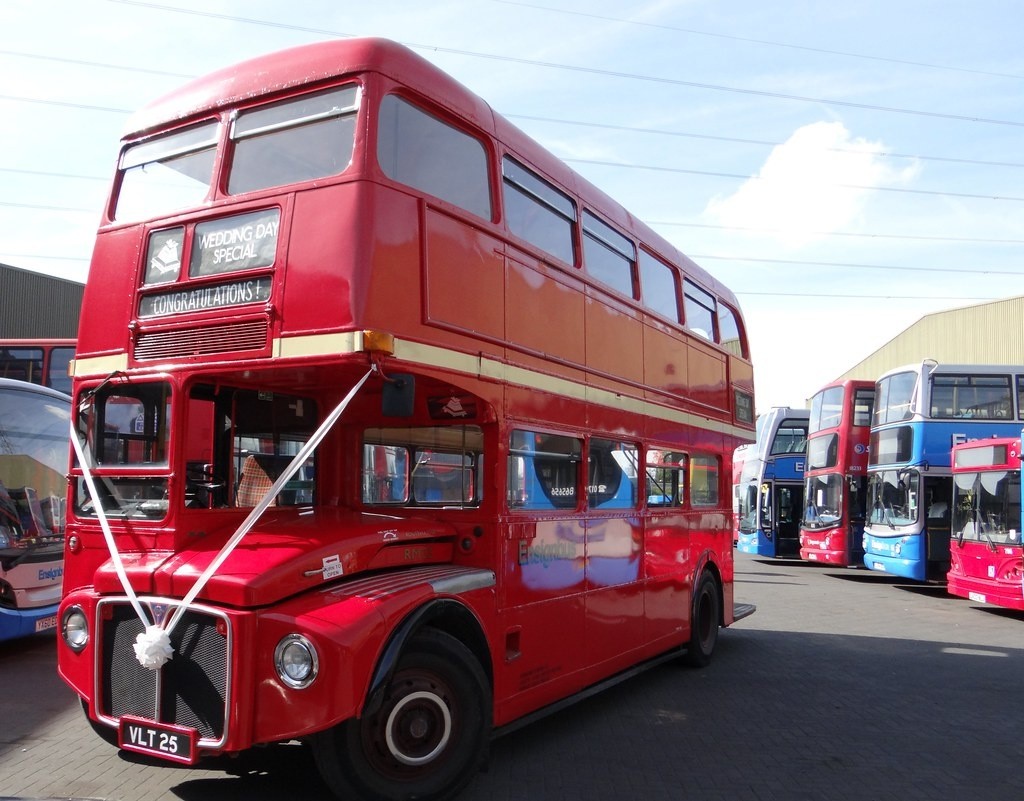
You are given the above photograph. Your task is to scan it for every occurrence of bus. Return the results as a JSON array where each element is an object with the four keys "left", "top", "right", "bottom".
[
  {"left": 0, "top": 37, "right": 757, "bottom": 801},
  {"left": 730, "top": 359, "right": 1022, "bottom": 613}
]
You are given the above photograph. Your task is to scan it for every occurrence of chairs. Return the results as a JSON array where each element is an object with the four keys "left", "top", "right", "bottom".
[
  {"left": 954, "top": 407, "right": 1004, "bottom": 420},
  {"left": 785, "top": 442, "right": 794, "bottom": 452},
  {"left": 233, "top": 453, "right": 300, "bottom": 508},
  {"left": 795, "top": 441, "right": 803, "bottom": 452},
  {"left": 798, "top": 440, "right": 807, "bottom": 453}
]
